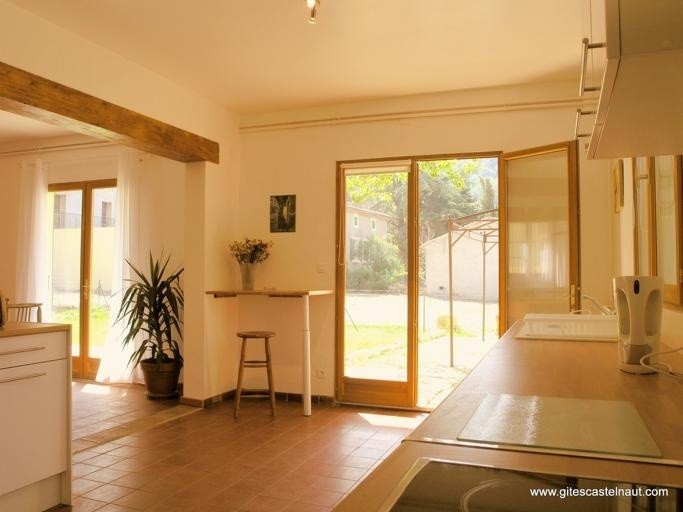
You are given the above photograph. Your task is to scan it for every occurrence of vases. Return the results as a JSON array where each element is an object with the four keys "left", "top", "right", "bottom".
[{"left": 239, "top": 263, "right": 257, "bottom": 289}]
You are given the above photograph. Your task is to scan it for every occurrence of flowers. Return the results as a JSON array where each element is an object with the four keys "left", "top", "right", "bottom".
[{"left": 228, "top": 238, "right": 273, "bottom": 264}]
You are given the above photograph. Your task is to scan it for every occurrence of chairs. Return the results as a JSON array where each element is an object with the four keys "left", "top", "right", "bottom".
[{"left": 5, "top": 298, "right": 43, "bottom": 323}]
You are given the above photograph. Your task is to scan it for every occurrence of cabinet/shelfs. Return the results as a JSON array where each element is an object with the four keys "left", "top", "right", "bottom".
[
  {"left": 629, "top": 155, "right": 683, "bottom": 305},
  {"left": 0, "top": 321, "right": 72, "bottom": 512},
  {"left": 574, "top": 0, "right": 683, "bottom": 160}
]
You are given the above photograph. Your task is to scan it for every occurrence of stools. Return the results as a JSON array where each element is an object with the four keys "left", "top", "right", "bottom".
[{"left": 234, "top": 330, "right": 277, "bottom": 417}]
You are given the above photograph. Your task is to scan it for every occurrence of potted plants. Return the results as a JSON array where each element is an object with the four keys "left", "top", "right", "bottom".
[{"left": 111, "top": 247, "right": 186, "bottom": 400}]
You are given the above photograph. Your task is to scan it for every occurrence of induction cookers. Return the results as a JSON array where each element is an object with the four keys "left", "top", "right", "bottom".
[{"left": 377, "top": 458, "right": 683, "bottom": 512}]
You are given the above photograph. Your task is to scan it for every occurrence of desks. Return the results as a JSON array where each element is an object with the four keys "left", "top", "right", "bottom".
[{"left": 205, "top": 288, "right": 333, "bottom": 416}]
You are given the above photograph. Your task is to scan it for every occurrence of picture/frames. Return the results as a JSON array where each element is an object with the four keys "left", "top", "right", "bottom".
[{"left": 613, "top": 159, "right": 623, "bottom": 213}]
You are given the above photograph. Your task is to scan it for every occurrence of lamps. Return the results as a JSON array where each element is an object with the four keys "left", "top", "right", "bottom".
[{"left": 305, "top": 0, "right": 320, "bottom": 24}]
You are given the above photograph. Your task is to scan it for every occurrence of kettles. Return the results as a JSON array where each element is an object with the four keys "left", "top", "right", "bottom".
[{"left": 612, "top": 275, "right": 662, "bottom": 376}]
